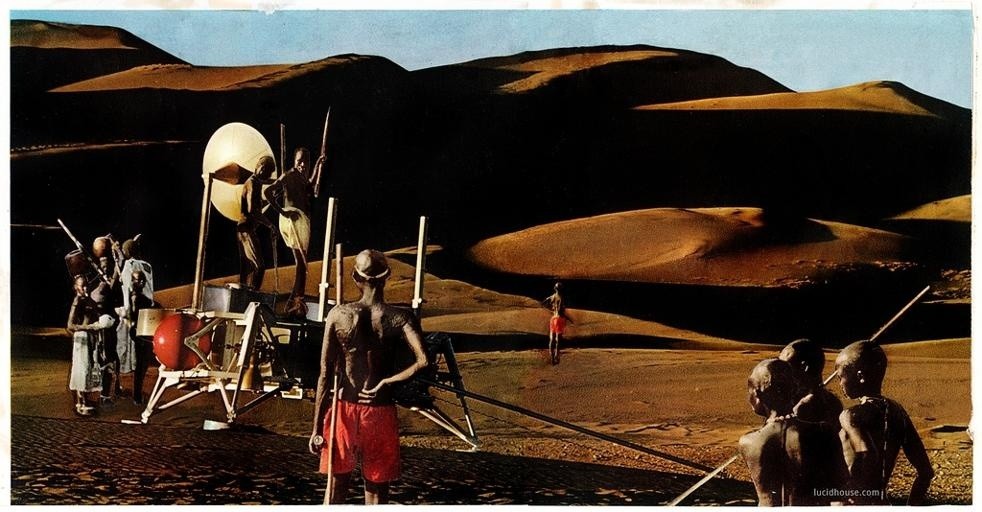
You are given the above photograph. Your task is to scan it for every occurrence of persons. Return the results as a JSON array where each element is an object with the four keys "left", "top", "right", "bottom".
[
  {"left": 67, "top": 235, "right": 161, "bottom": 419},
  {"left": 778, "top": 338, "right": 842, "bottom": 434},
  {"left": 737, "top": 358, "right": 846, "bottom": 505},
  {"left": 305, "top": 247, "right": 434, "bottom": 504},
  {"left": 830, "top": 339, "right": 934, "bottom": 505},
  {"left": 235, "top": 152, "right": 279, "bottom": 294},
  {"left": 264, "top": 146, "right": 322, "bottom": 299},
  {"left": 540, "top": 281, "right": 573, "bottom": 365}
]
